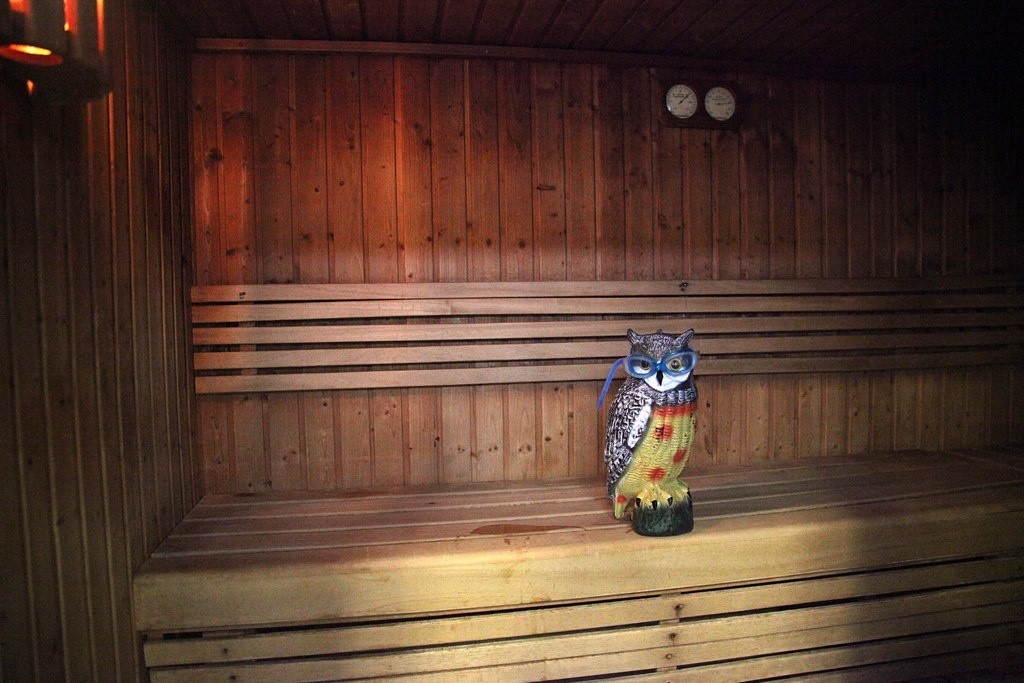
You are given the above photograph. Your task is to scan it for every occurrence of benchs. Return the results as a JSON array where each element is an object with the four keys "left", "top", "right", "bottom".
[{"left": 131, "top": 277, "right": 1024, "bottom": 683}]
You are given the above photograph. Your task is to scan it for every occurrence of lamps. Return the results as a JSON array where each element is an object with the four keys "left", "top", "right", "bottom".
[{"left": 0, "top": 0, "right": 67, "bottom": 66}]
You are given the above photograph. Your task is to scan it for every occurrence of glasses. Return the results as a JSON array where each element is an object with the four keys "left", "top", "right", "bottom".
[{"left": 597, "top": 351, "right": 698, "bottom": 409}]
